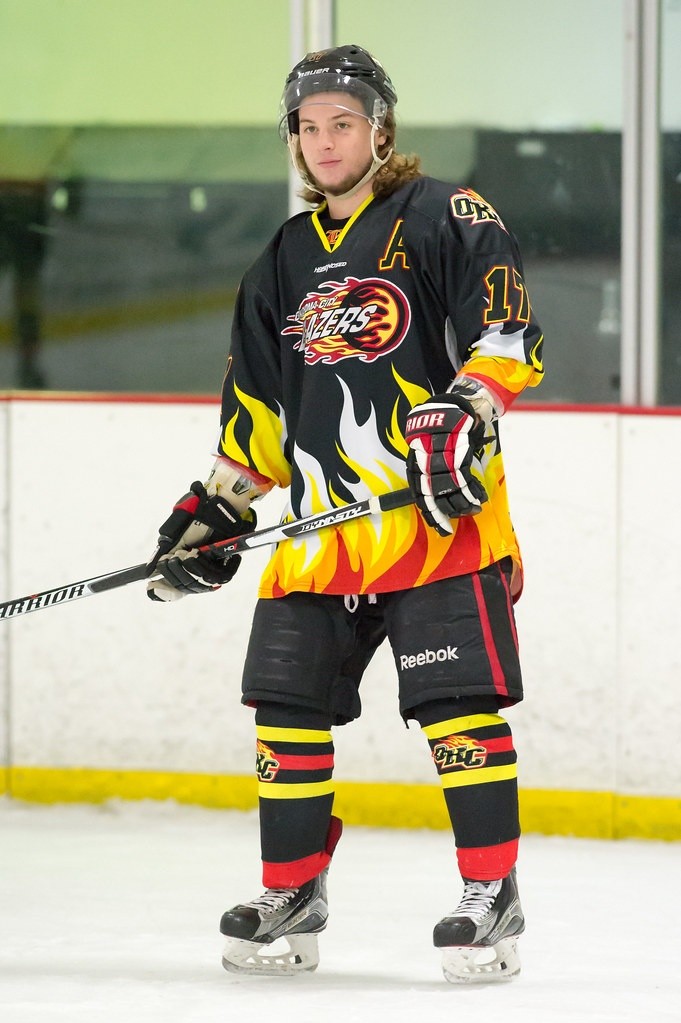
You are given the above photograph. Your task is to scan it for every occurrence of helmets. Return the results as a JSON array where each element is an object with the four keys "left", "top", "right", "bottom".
[{"left": 284, "top": 44, "right": 398, "bottom": 134}]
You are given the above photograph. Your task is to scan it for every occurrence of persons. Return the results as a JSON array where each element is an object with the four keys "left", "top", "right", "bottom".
[{"left": 148, "top": 43, "right": 546, "bottom": 947}]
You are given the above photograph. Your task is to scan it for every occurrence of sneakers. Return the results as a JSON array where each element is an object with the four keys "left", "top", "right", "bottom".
[
  {"left": 220, "top": 817, "right": 343, "bottom": 976},
  {"left": 433, "top": 868, "right": 527, "bottom": 983}
]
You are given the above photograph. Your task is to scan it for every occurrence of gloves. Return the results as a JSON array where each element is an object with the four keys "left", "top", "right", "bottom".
[
  {"left": 404, "top": 391, "right": 488, "bottom": 539},
  {"left": 145, "top": 480, "right": 258, "bottom": 603}
]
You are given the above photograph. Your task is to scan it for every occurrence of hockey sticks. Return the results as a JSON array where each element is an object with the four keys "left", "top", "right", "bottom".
[{"left": 0, "top": 485, "right": 416, "bottom": 624}]
